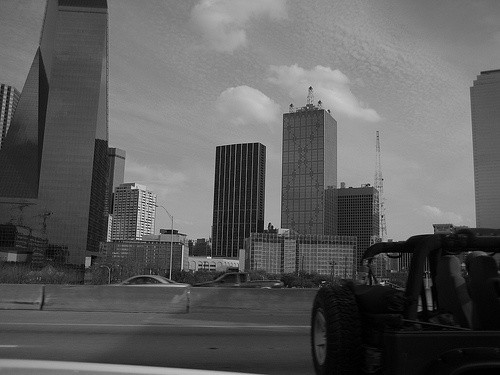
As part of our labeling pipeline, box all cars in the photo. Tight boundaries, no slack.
[118,273,192,287]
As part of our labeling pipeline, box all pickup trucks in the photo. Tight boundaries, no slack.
[195,270,285,288]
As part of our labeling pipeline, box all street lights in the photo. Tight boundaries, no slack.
[100,264,119,284]
[153,204,174,281]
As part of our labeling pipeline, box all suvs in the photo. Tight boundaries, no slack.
[310,223,499,375]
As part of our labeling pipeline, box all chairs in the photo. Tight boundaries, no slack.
[451,255,500,332]
